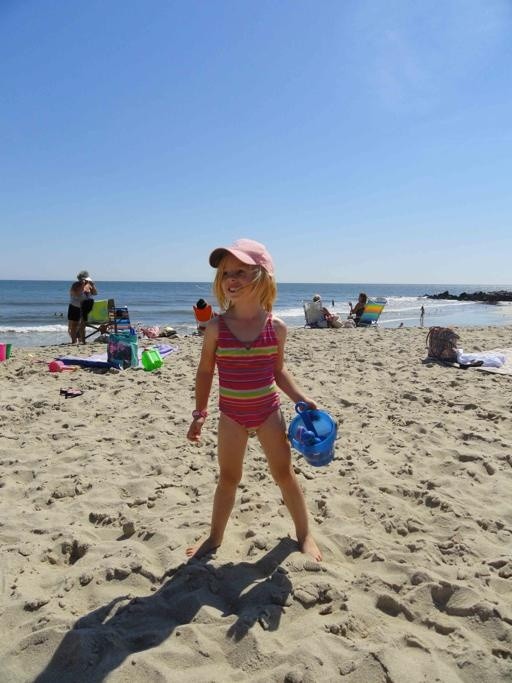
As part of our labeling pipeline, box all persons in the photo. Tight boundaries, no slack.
[186,239,321,563]
[312,293,367,323]
[196,298,208,309]
[420,305,425,320]
[67,270,98,344]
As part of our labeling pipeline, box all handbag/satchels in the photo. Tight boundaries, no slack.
[426,325,459,363]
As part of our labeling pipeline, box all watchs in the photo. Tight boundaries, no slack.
[192,410,208,418]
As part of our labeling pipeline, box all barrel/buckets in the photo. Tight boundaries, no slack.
[286,409,340,468]
[0,342,7,361]
[6,344,13,359]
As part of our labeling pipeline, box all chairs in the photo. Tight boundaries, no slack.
[355,300,385,327]
[193,304,215,331]
[80,296,131,340]
[301,300,330,327]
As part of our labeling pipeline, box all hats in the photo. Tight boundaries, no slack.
[76,270,92,283]
[209,239,274,276]
